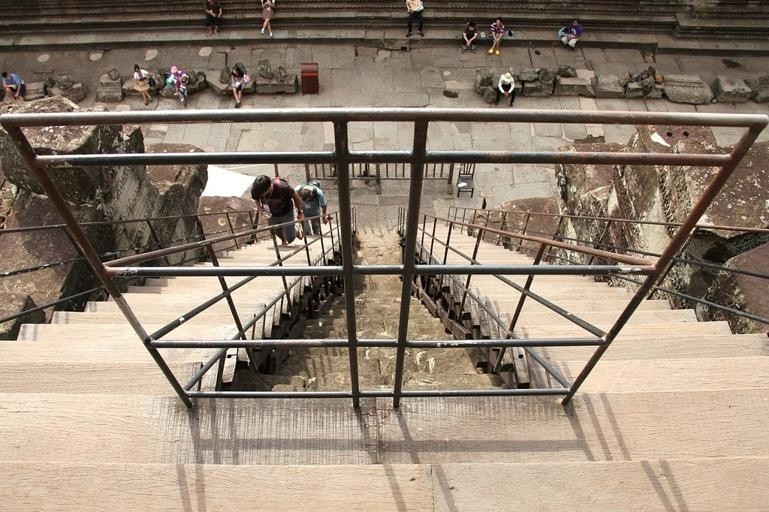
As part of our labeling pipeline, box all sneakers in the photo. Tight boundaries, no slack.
[235,100,241,107]
[145,96,152,104]
[259,30,273,39]
[488,48,501,55]
[295,224,303,240]
[406,31,424,37]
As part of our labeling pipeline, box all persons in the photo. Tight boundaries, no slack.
[1,71,26,101]
[493,71,516,107]
[294,183,328,237]
[175,71,188,97]
[203,0,223,37]
[165,65,189,108]
[560,18,585,53]
[488,16,505,57]
[557,25,578,48]
[231,65,245,109]
[260,0,276,38]
[250,174,305,247]
[133,63,153,106]
[461,18,479,54]
[406,0,425,38]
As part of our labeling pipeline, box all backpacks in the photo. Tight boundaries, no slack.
[272,177,289,195]
[298,181,321,200]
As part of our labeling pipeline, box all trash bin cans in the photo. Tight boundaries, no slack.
[301,63,318,94]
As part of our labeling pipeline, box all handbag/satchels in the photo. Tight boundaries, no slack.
[243,74,252,83]
[149,77,156,87]
[165,76,174,88]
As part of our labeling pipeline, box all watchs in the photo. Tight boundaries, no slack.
[297,208,304,214]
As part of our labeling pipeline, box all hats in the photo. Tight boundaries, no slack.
[171,65,177,72]
